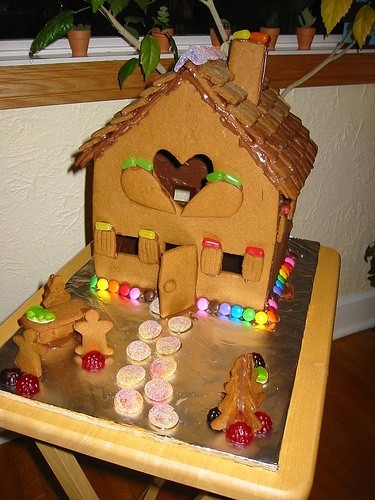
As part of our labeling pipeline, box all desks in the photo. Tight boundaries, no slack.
[18,296,89,345]
[0,238,342,500]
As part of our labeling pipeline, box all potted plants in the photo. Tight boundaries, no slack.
[296,7,317,50]
[260,11,280,51]
[151,6,174,54]
[210,18,231,49]
[67,23,92,57]
